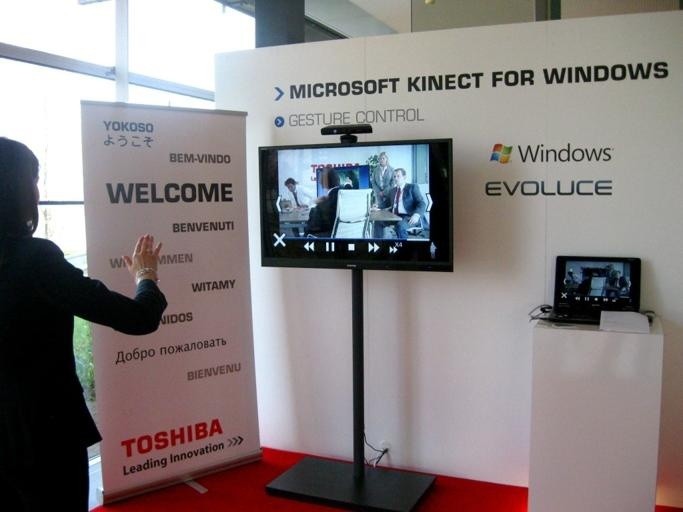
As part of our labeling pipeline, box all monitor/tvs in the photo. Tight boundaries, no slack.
[257,139,455,274]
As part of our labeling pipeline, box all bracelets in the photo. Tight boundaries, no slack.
[134,266,160,287]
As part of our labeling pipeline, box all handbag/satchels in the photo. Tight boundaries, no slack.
[308,208,333,232]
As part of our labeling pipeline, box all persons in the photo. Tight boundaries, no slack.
[563,265,629,298]
[370,151,395,210]
[279,178,317,238]
[0,135,167,512]
[305,167,344,237]
[373,168,426,240]
[342,170,358,188]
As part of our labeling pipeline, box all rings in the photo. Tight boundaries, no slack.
[145,248,152,254]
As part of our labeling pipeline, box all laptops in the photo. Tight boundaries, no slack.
[547,255,642,326]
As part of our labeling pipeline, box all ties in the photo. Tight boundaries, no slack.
[293,191,300,207]
[394,188,400,214]
